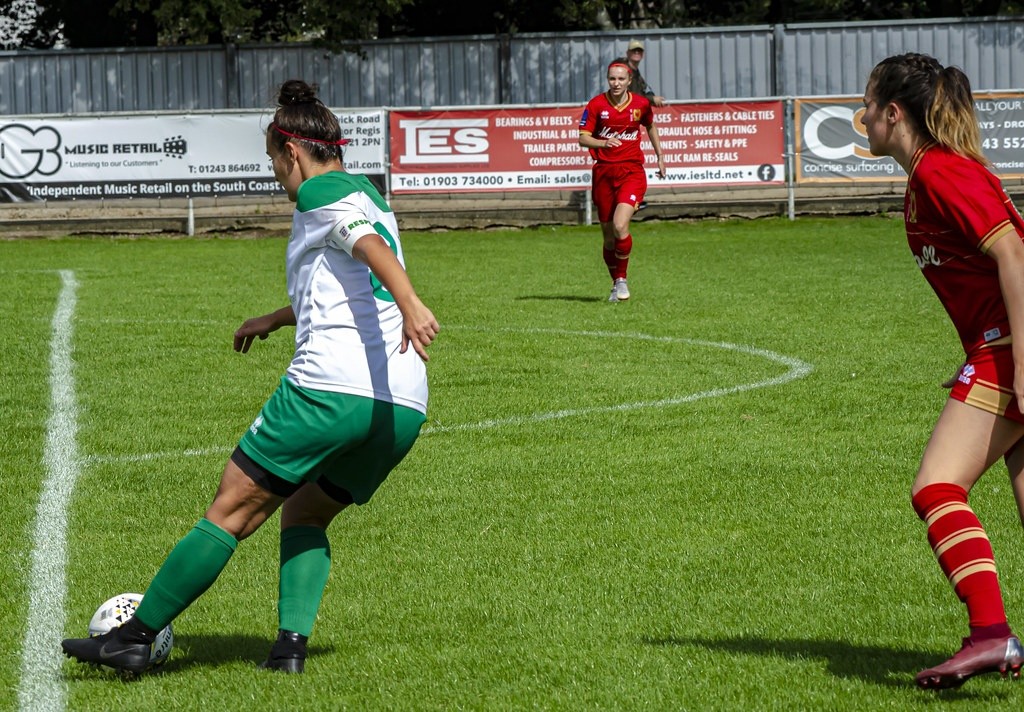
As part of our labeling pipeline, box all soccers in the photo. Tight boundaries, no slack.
[90,593,174,667]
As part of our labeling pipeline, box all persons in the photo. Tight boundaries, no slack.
[59,81,443,684]
[577,58,669,306]
[859,52,1024,692]
[623,42,667,106]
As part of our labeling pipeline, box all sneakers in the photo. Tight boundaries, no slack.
[61,624,151,682]
[258,628,310,674]
[609,286,620,302]
[615,277,630,299]
[913,635,1024,691]
